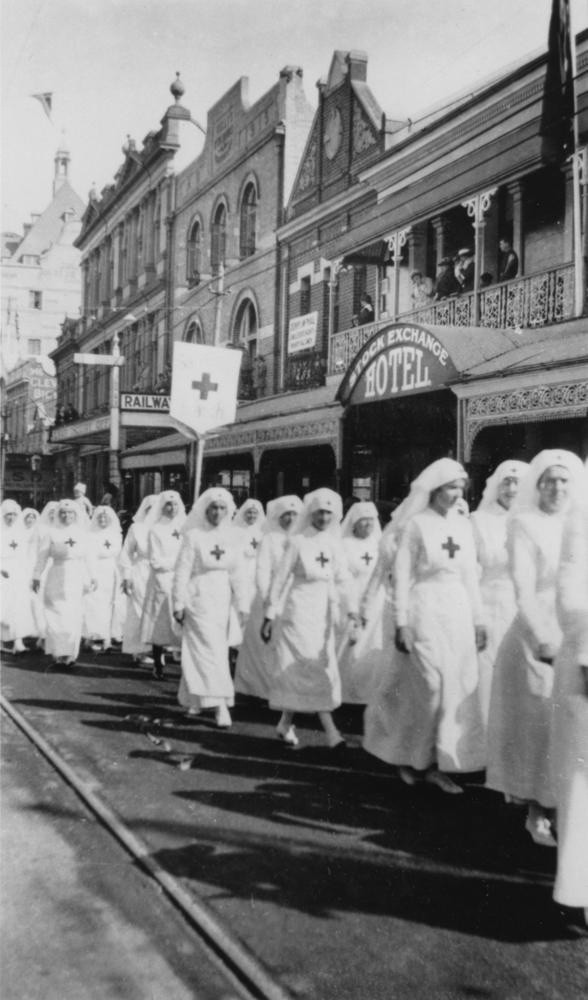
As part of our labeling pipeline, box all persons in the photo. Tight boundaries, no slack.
[51,235,518,427]
[0,447,588,915]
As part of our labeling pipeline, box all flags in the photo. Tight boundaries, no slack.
[539,1,572,176]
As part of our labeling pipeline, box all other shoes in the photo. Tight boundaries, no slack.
[400,770,415,785]
[132,654,154,664]
[275,723,298,744]
[328,732,347,751]
[13,647,29,655]
[524,812,557,848]
[171,654,181,662]
[103,642,112,651]
[85,641,101,651]
[423,773,465,795]
[160,653,166,666]
[188,708,201,714]
[152,667,164,681]
[215,707,232,727]
